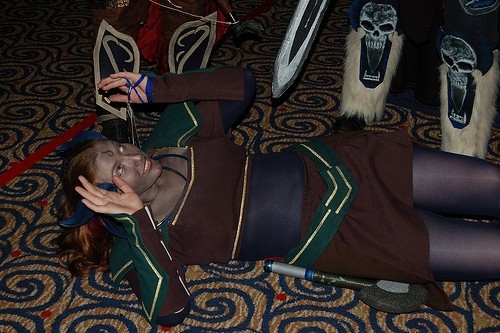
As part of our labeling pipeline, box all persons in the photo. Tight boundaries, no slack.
[55,69,500,323]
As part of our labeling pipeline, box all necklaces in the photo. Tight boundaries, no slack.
[152,152,190,182]
